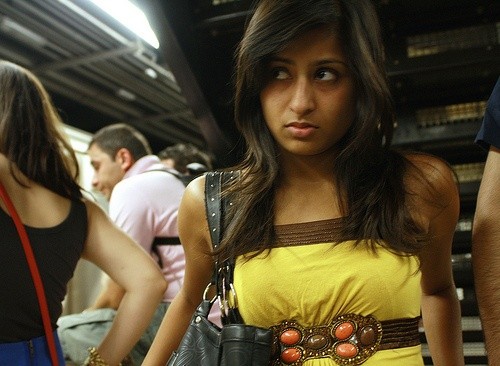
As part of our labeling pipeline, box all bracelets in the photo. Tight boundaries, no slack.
[87,346,123,366]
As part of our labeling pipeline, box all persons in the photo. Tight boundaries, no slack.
[81,124,224,366]
[140,0,465,366]
[0,58,167,366]
[472,78,500,366]
[158,143,212,177]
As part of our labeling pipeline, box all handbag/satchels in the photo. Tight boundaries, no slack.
[168,168,276,363]
